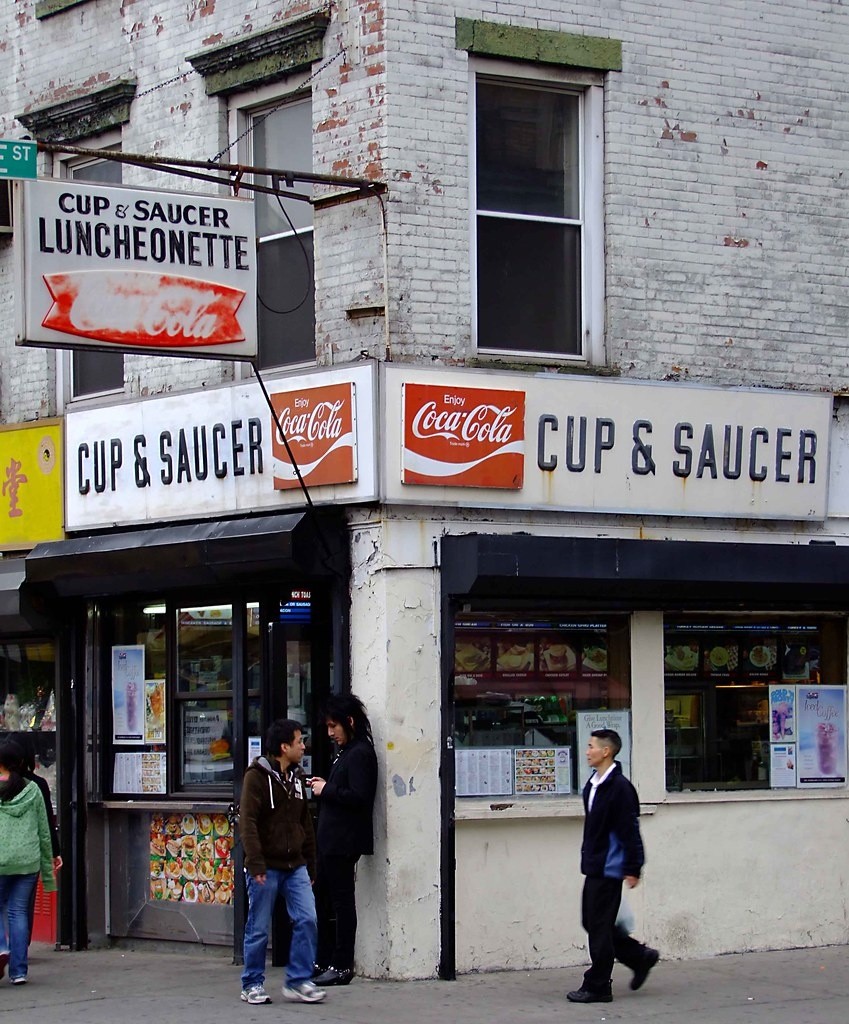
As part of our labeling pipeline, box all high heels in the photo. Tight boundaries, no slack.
[311,965,354,984]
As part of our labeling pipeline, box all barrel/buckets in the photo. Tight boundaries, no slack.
[454,684,477,699]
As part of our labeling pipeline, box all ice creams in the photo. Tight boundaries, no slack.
[776,702,788,739]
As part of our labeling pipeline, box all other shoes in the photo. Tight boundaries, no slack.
[11,977,28,983]
[0,952,10,980]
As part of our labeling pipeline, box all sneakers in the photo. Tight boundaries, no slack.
[241,985,272,1004]
[282,980,326,1001]
[567,986,613,1003]
[630,949,659,990]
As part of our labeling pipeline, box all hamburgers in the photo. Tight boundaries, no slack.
[549,644,567,668]
[462,647,481,664]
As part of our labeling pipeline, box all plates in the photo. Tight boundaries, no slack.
[182,813,229,836]
[709,647,729,667]
[583,647,607,672]
[749,645,771,667]
[166,841,214,902]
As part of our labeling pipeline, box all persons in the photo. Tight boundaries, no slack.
[306,693,378,984]
[0,731,63,983]
[241,720,327,1003]
[568,730,659,1003]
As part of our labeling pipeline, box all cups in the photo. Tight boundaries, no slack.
[817,722,839,777]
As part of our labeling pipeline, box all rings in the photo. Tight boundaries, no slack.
[312,789,315,791]
